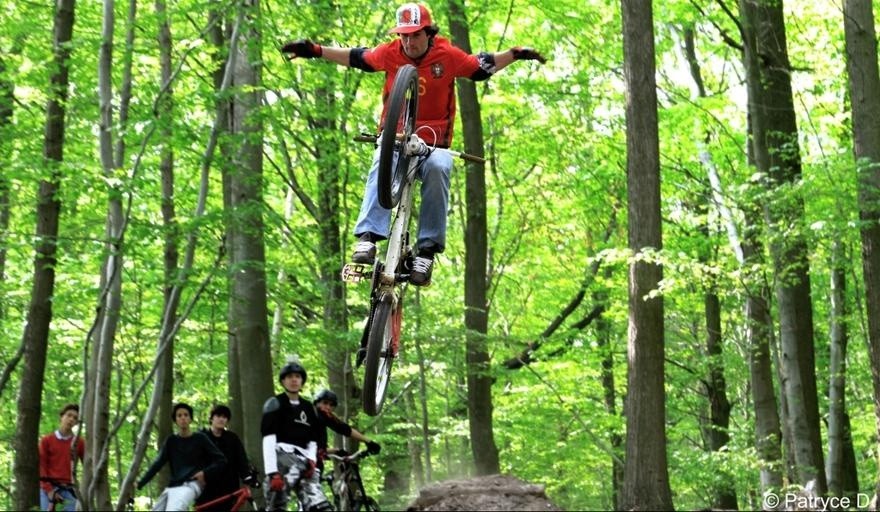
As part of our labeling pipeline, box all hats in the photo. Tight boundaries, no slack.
[388,3,432,34]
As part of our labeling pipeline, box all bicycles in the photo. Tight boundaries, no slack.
[39,472,82,511]
[193,466,265,510]
[341,67,488,416]
[262,444,382,512]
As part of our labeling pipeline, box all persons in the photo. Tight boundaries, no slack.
[197,403,251,505]
[281,2,548,285]
[257,361,335,512]
[311,388,379,484]
[40,403,85,512]
[130,402,227,511]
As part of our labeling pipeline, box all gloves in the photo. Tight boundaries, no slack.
[270,473,288,490]
[334,448,352,459]
[316,452,325,472]
[512,46,546,65]
[364,440,380,455]
[282,39,322,60]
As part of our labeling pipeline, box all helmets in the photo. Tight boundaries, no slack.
[313,389,337,408]
[281,362,306,386]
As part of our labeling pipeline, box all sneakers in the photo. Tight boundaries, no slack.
[352,232,377,264]
[408,249,434,286]
